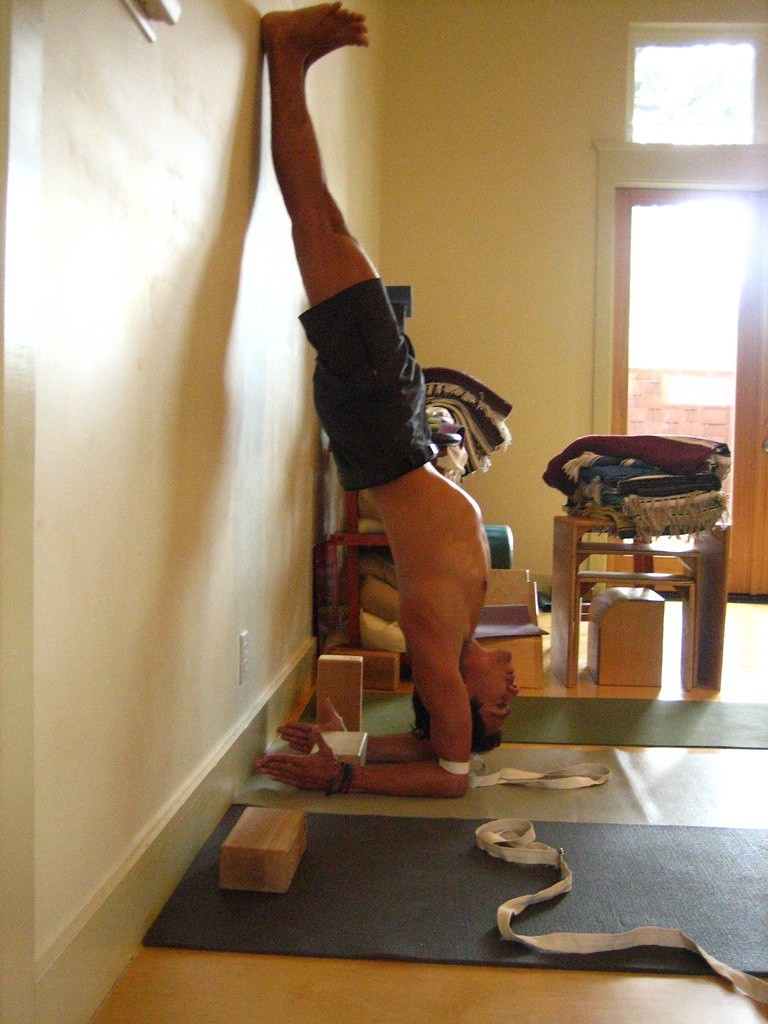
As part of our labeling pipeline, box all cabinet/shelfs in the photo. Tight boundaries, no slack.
[328,441,446,665]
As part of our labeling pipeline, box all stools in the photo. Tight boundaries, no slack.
[575,572,696,691]
[550,515,731,692]
[571,541,704,689]
[588,585,665,688]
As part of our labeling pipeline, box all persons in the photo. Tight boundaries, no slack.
[257,2,517,798]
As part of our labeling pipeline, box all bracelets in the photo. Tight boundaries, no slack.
[318,761,353,797]
